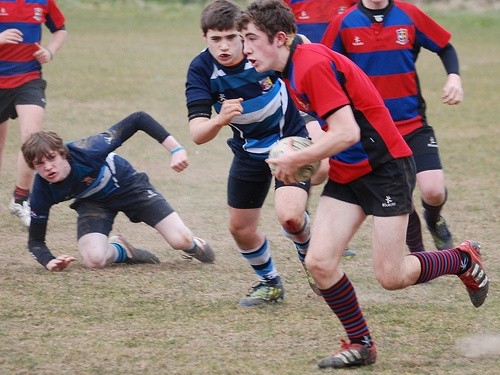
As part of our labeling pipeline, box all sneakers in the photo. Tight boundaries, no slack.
[185,239,215,263]
[8,197,31,227]
[240,276,284,306]
[423,211,453,252]
[299,258,323,297]
[342,249,355,259]
[454,240,489,308]
[108,235,160,265]
[317,339,376,369]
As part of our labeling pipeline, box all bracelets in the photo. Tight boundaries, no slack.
[47,48,53,60]
[169,146,185,154]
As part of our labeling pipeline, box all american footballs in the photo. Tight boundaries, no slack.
[268,136,320,182]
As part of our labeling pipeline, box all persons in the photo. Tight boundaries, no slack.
[235,0,489,369]
[282,0,357,186]
[185,0,322,307]
[21,111,215,271]
[321,0,463,253]
[0,0,67,229]
